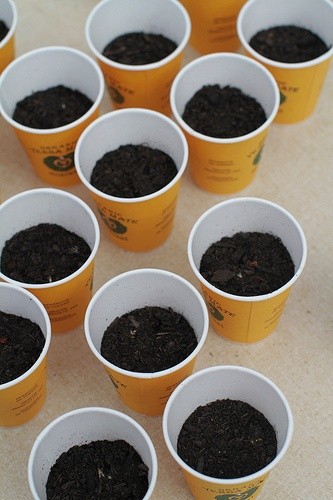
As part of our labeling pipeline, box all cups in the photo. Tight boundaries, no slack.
[236,0,333,125]
[161,366,293,500]
[84,269,209,416]
[178,0,252,55]
[0,283,51,426]
[0,46,103,188]
[170,52,280,194]
[0,189,100,334]
[0,0,18,75]
[74,109,189,253]
[28,407,158,500]
[187,197,307,344]
[86,0,191,118]
